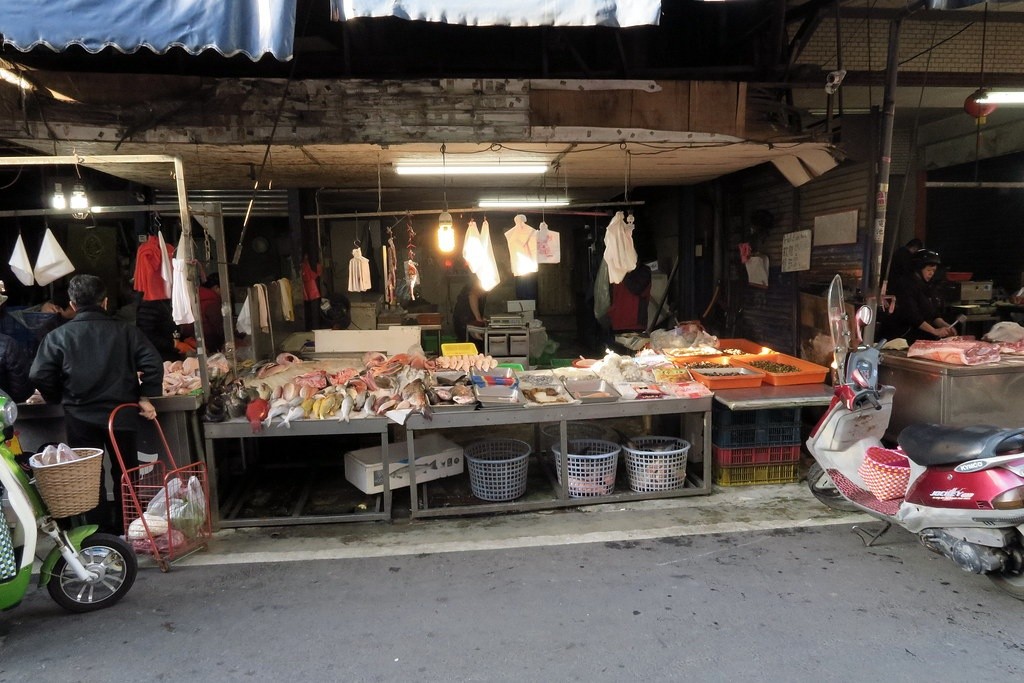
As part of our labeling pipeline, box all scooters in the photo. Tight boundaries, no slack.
[804,274,1023,604]
[0,386,139,615]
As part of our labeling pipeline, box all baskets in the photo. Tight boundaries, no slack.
[29,447,104,518]
[462,438,531,501]
[542,423,690,500]
[857,445,912,501]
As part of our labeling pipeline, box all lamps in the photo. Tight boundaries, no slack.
[392,162,551,176]
[975,0,1024,105]
[476,197,571,208]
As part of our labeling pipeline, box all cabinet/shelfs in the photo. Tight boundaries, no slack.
[466,320,529,370]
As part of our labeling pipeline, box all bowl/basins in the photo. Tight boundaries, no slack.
[572,358,596,367]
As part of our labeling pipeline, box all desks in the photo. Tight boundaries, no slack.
[405,347,714,519]
[202,355,391,532]
[416,325,442,358]
[614,332,835,467]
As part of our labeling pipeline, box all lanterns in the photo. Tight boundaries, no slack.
[963,89,996,125]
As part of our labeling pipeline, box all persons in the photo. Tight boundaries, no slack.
[452,282,488,350]
[885,239,956,346]
[112,254,247,360]
[26,275,164,534]
[0,276,78,405]
[608,264,652,339]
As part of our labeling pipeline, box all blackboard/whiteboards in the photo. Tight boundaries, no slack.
[781,229,811,272]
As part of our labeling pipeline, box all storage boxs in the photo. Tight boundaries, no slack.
[943,281,994,301]
[712,406,803,427]
[711,442,800,467]
[345,432,464,494]
[711,460,800,486]
[518,311,534,321]
[346,294,384,330]
[507,300,535,313]
[712,423,801,448]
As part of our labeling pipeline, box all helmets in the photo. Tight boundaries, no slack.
[914,249,941,271]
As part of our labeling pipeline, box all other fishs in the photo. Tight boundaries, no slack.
[226,375,476,434]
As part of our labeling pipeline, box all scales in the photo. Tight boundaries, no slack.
[491,313,523,329]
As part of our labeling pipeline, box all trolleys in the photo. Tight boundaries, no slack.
[109,403,212,574]
